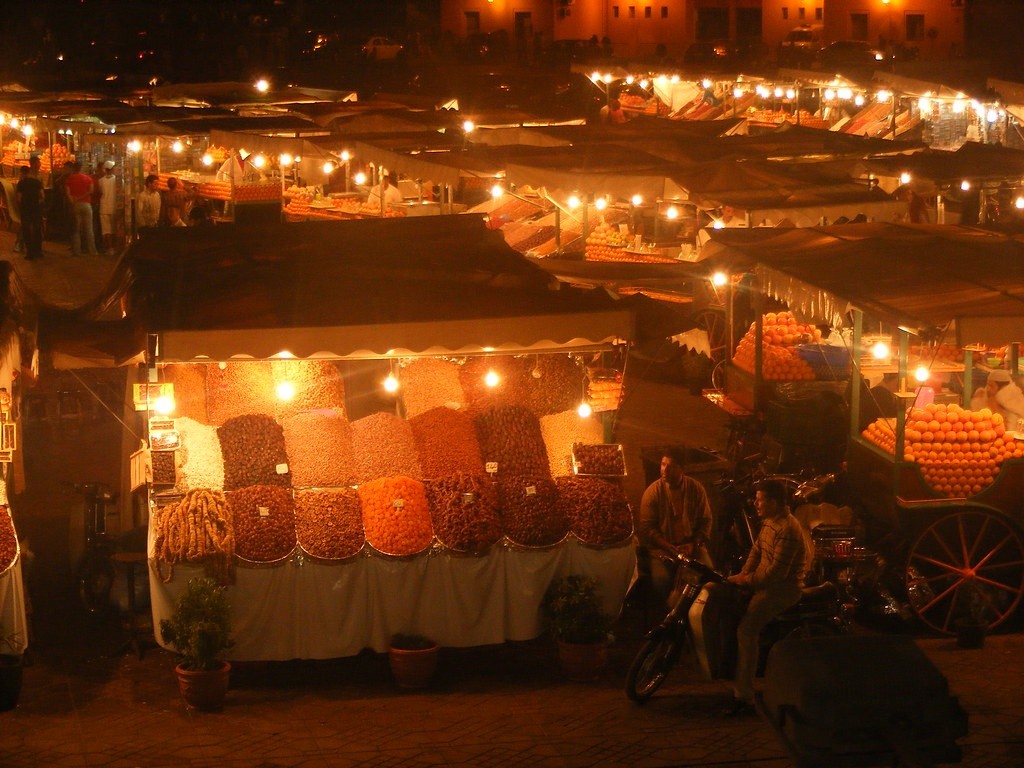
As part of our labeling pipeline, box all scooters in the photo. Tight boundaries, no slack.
[624,552,845,704]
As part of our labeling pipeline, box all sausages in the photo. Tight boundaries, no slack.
[151,487,236,589]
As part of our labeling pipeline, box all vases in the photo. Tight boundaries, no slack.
[0,655,25,710]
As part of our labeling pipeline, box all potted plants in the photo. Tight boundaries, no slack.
[160,575,231,711]
[954,577,988,647]
[552,573,613,679]
[386,633,439,688]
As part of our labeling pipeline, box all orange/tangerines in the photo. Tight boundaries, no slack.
[862,403,1024,498]
[584,245,681,263]
[733,311,825,381]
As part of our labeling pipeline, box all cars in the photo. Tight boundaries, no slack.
[816,40,896,66]
[683,41,736,67]
[351,32,405,61]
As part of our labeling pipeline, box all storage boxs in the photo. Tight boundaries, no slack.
[797,344,850,367]
[950,369,989,393]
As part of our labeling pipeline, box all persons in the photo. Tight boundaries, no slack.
[599,99,626,123]
[640,448,815,716]
[0,135,258,259]
[972,369,1018,433]
[708,203,747,228]
[695,84,720,107]
[861,372,904,428]
[369,164,403,205]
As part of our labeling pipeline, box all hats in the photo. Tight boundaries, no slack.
[167,177,177,190]
[104,159,116,170]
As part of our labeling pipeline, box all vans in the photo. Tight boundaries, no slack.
[779,24,823,57]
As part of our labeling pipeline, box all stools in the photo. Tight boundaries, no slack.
[111,552,158,661]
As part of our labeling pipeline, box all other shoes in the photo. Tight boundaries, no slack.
[721,698,744,716]
